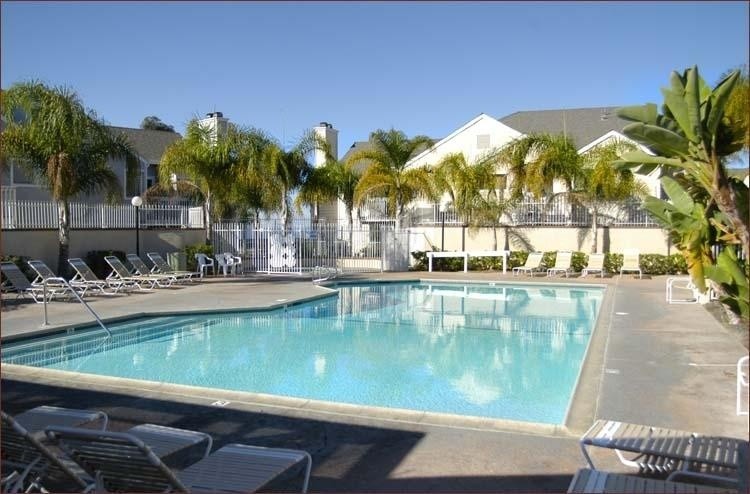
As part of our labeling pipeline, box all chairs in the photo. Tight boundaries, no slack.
[3,406,110,489]
[0,411,214,491]
[43,418,312,493]
[513,249,643,279]
[0,252,242,303]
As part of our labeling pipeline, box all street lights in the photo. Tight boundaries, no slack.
[131,196,142,256]
[439,205,448,251]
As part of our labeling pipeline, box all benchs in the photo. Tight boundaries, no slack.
[559,419,749,494]
